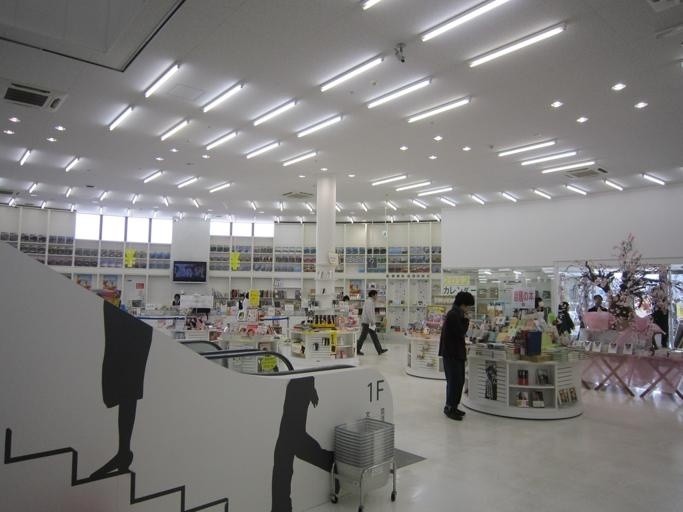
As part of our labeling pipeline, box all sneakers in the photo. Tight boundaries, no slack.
[356,350,364,355]
[454,408,465,416]
[442,406,463,421]
[377,348,389,355]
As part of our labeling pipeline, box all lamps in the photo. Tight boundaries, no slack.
[7,0,667,223]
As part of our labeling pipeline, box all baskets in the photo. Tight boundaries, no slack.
[334,416,395,495]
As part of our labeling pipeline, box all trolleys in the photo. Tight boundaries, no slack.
[328,458,399,512]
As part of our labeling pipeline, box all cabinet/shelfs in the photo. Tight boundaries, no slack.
[1,230,682,421]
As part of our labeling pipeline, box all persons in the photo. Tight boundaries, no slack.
[588,295,607,312]
[355,290,388,355]
[532,297,548,325]
[556,302,575,335]
[439,291,475,420]
[173,294,180,306]
[242,292,249,319]
[647,307,678,348]
[238,294,245,309]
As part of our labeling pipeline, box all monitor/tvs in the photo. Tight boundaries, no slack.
[173,261,207,283]
